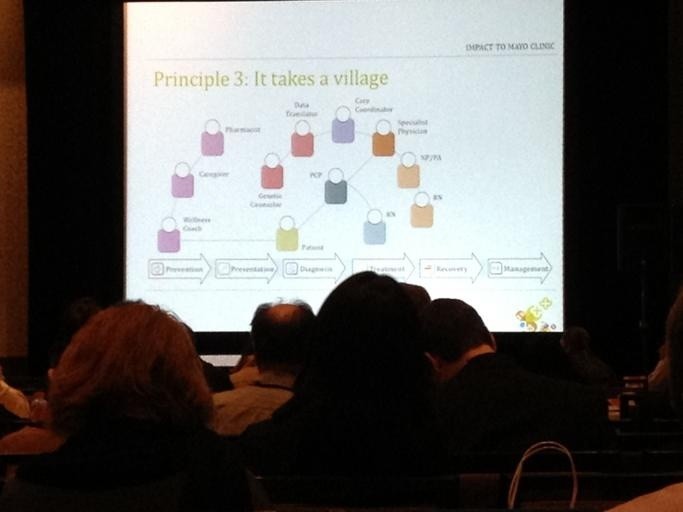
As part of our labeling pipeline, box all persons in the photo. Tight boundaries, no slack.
[1,268,681,511]
